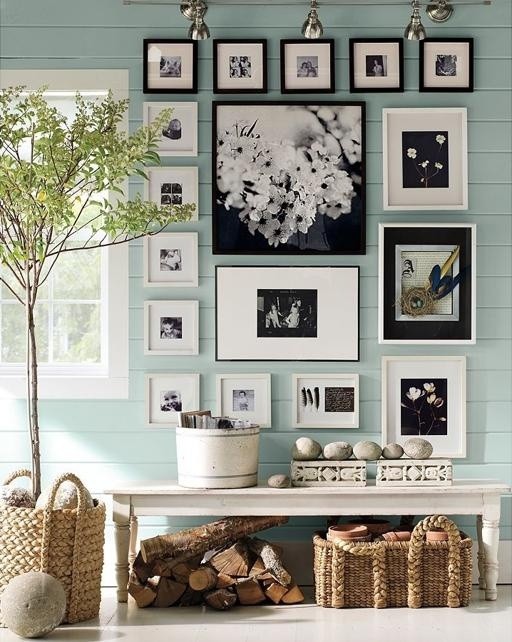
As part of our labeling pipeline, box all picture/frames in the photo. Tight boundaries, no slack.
[280,38,334,94]
[213,39,267,93]
[349,37,404,93]
[143,101,198,157]
[378,222,476,344]
[215,265,360,362]
[382,108,468,210]
[143,38,197,94]
[217,374,271,429]
[381,355,466,458]
[292,374,359,428]
[143,231,198,288]
[212,101,366,254]
[418,37,473,92]
[144,300,200,355]
[143,373,200,429]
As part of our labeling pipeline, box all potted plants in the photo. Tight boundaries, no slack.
[0,84,198,624]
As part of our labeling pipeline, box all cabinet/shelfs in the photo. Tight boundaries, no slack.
[106,479,512,602]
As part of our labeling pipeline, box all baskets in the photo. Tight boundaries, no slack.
[0,472,107,629]
[312,515,474,609]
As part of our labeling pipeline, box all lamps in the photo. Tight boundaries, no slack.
[403,15,426,40]
[189,14,210,40]
[301,12,325,39]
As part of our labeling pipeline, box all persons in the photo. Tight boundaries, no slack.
[371,59,383,77]
[238,390,250,412]
[286,305,301,328]
[161,320,179,338]
[159,390,182,413]
[161,119,182,140]
[297,61,315,77]
[230,56,252,78]
[270,304,283,328]
